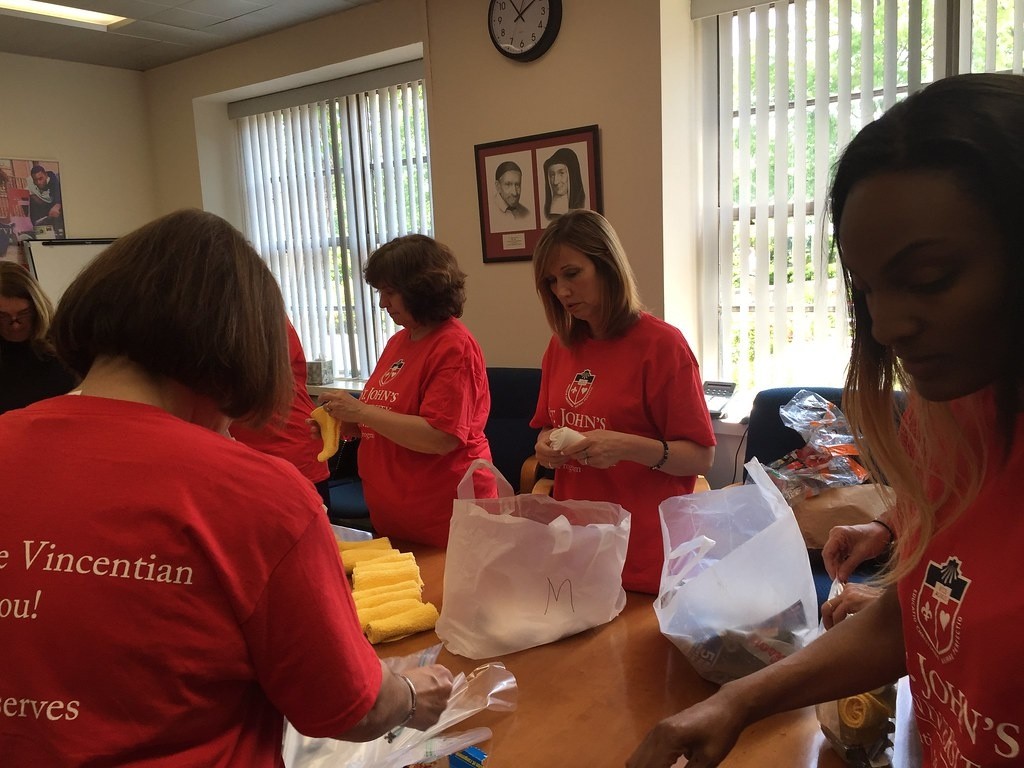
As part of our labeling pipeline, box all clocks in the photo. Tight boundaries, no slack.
[488,0,563,62]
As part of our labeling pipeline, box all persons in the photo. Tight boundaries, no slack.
[225,314,332,526]
[625,74,1024,768]
[531,208,718,601]
[306,234,500,552]
[821,507,899,632]
[0,261,82,416]
[0,207,458,767]
[21,164,64,238]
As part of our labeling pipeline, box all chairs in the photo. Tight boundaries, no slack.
[723,387,904,624]
[484,366,554,496]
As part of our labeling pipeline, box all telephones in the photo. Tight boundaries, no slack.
[701,380,736,419]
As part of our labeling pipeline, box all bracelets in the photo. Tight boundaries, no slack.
[871,520,896,548]
[384,672,416,744]
[650,440,669,470]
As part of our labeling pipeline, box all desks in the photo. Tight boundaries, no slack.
[332,522,923,768]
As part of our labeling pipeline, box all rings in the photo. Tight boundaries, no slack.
[826,601,836,611]
[327,402,332,411]
[585,457,588,465]
[548,461,552,469]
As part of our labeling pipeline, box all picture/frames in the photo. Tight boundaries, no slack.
[473,123,602,264]
